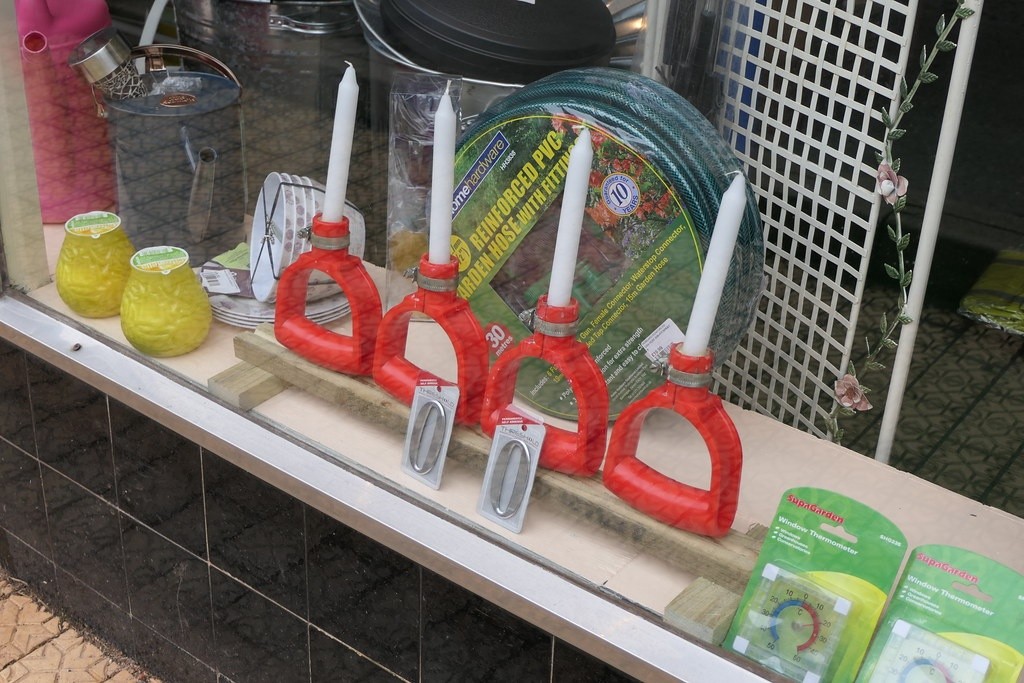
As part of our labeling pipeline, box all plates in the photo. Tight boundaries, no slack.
[204,289,355,332]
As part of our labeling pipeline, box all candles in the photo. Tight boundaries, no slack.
[428,81,456,267]
[321,60,360,221]
[546,119,593,308]
[681,170,747,356]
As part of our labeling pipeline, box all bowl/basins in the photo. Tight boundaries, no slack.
[248,170,366,306]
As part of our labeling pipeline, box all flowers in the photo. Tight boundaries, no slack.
[824,0,975,446]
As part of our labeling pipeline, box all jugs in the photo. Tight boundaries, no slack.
[69,26,247,266]
